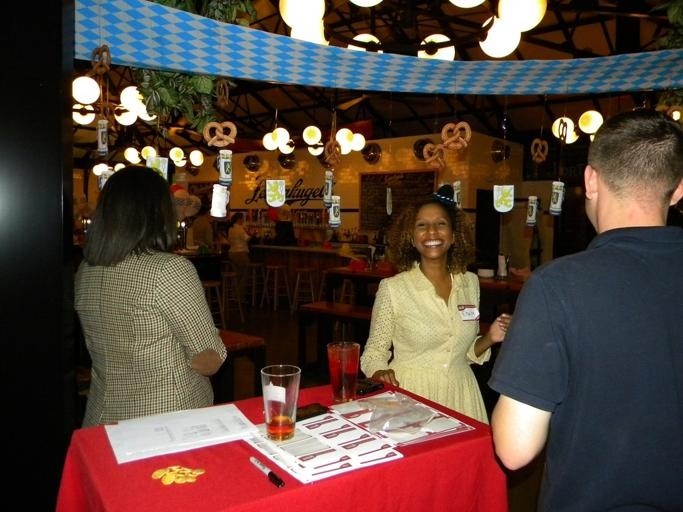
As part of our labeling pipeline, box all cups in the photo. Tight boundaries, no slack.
[326,341,361,403]
[260,365,302,441]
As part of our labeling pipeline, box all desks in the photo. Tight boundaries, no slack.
[321,260,527,315]
[51,376,509,511]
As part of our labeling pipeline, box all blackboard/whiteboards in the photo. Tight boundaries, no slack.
[358,169,438,230]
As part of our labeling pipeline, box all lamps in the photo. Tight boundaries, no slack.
[75,0,602,180]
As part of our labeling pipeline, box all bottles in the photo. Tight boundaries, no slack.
[340,227,359,242]
[296,211,320,224]
[496,255,507,280]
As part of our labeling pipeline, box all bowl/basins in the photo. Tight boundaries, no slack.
[477,269,494,278]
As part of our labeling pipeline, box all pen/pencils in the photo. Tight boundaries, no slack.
[250,457,285,488]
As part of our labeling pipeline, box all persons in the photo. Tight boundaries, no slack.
[226,212,252,304]
[489,106,683,512]
[361,186,512,426]
[73,164,228,428]
[270,204,298,246]
[192,206,213,245]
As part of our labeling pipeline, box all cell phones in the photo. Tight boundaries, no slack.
[358,382,386,395]
[297,403,325,421]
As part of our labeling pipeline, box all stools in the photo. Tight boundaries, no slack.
[294,298,490,366]
[201,257,354,332]
[208,329,267,404]
